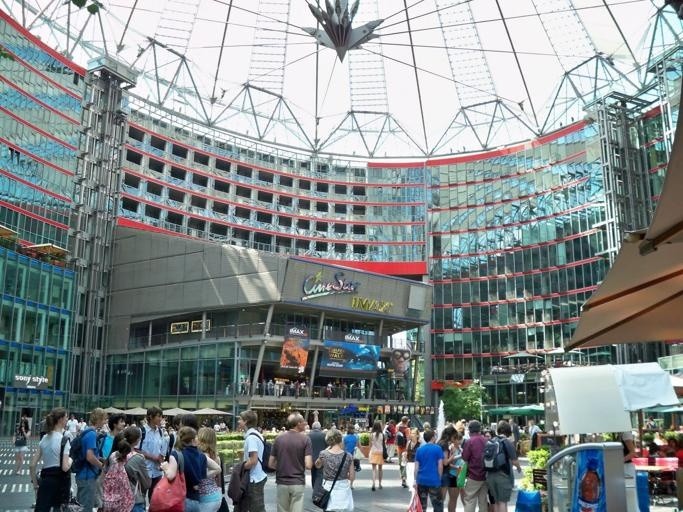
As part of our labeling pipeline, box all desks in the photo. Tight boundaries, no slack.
[634,465,671,505]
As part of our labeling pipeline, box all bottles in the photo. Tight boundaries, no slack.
[578,459,602,512]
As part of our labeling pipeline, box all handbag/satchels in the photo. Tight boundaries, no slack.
[312,480,330,510]
[383,445,388,459]
[217,497,230,512]
[150,471,187,512]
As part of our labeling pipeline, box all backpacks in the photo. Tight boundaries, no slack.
[69,436,83,473]
[481,438,502,472]
[101,460,136,512]
[262,439,276,474]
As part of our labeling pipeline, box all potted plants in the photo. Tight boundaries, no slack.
[358,432,371,459]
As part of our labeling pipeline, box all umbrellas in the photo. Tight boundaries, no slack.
[486,404,545,426]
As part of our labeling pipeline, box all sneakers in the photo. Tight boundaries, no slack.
[16,472,22,475]
[402,482,408,488]
[378,486,383,489]
[372,487,376,491]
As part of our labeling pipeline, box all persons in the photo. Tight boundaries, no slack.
[313,427,355,511]
[332,410,545,463]
[307,420,326,487]
[11,418,30,476]
[619,409,683,491]
[228,408,267,511]
[390,340,411,373]
[267,412,313,511]
[280,327,308,377]
[30,405,234,511]
[369,424,385,492]
[224,374,407,401]
[395,416,521,511]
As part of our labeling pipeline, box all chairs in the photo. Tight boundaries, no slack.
[655,456,679,497]
[631,458,649,466]
